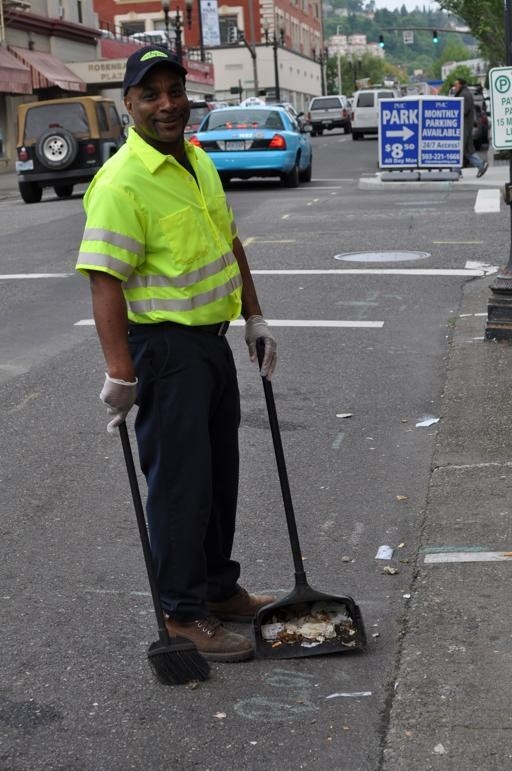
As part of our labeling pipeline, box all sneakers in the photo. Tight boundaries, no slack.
[476,162,489,178]
[459,172,463,179]
[202,585,276,626]
[162,610,255,665]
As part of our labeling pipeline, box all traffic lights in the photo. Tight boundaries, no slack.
[433,31,438,44]
[379,35,384,48]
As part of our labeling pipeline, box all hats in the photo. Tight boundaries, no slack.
[121,43,190,97]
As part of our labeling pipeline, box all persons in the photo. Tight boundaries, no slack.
[452,78,489,178]
[76,42,280,665]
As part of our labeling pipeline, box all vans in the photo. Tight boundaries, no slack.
[448,86,486,107]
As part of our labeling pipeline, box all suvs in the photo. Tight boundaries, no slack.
[15,95,130,204]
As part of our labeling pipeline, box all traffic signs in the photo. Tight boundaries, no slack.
[379,97,465,169]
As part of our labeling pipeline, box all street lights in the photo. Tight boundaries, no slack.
[336,25,344,95]
[311,40,329,96]
[262,16,286,101]
[347,55,363,90]
[162,0,193,66]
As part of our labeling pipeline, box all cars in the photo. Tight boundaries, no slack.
[185,89,399,187]
[472,106,487,151]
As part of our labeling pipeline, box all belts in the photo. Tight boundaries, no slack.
[128,320,231,339]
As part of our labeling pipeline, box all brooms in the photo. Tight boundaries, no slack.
[117,420,210,687]
